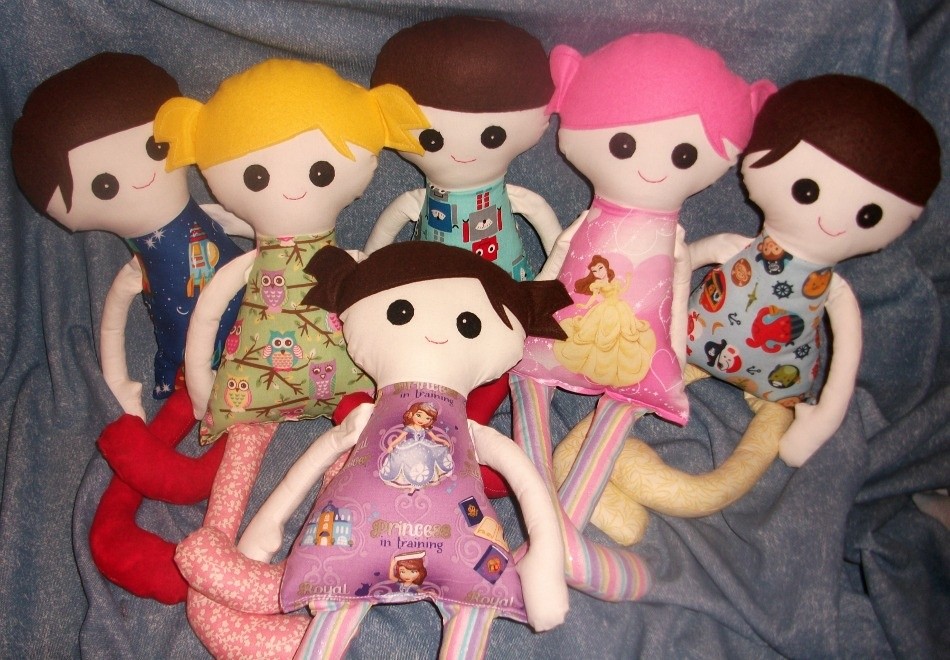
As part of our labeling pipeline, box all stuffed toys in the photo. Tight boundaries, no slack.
[365,10,566,287]
[9,49,255,607]
[505,29,782,607]
[234,238,573,660]
[149,55,433,660]
[548,70,945,547]
